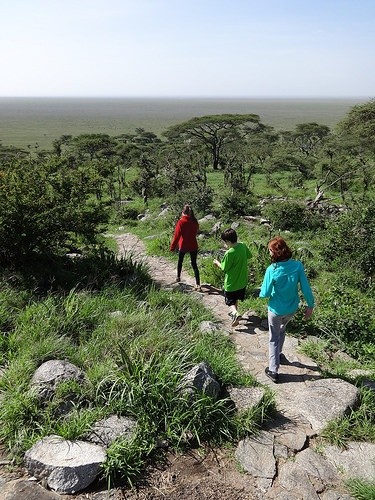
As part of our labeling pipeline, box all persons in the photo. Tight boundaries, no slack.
[169,205,202,292]
[213,228,252,327]
[259,236,314,381]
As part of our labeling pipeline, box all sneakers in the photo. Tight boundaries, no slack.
[192,286,202,291]
[174,278,180,282]
[230,311,242,327]
[228,312,234,317]
[264,367,279,383]
